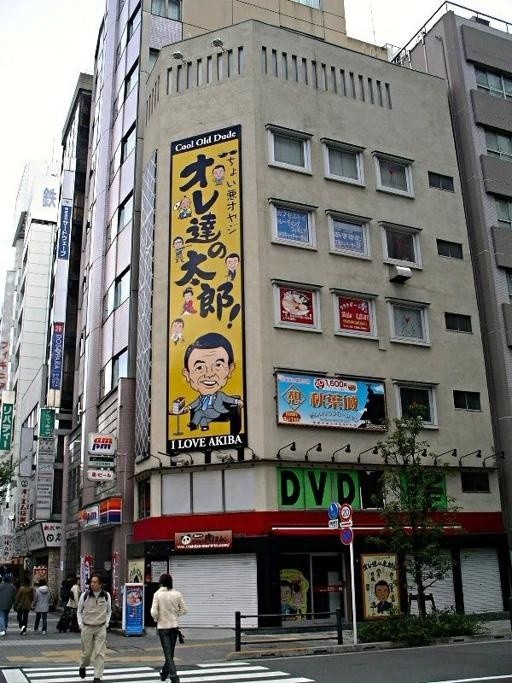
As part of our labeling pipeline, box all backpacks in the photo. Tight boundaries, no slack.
[62,584,74,602]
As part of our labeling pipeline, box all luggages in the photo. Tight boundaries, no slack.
[57,614,71,633]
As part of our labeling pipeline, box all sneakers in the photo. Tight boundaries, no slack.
[21,626,26,635]
[0,631,6,636]
[34,630,46,635]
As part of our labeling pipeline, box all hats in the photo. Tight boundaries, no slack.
[4,576,13,583]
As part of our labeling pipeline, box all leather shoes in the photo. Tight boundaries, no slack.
[79,668,85,678]
[160,669,166,681]
[93,680,102,683]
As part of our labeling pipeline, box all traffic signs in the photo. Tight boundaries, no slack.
[339,504,352,521]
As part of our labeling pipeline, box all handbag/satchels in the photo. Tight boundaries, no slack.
[178,630,184,644]
[14,602,23,612]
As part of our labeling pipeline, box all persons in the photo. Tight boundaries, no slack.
[0,566,21,590]
[149,573,188,683]
[13,577,33,635]
[58,575,82,633]
[32,579,51,636]
[0,577,18,637]
[77,573,112,683]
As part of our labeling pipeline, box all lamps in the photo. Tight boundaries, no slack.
[210,38,226,52]
[172,52,186,65]
[277,442,505,468]
[142,441,256,468]
